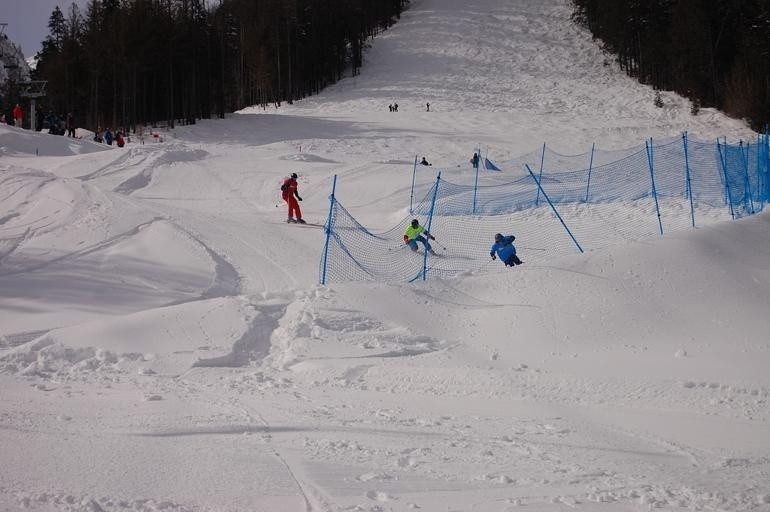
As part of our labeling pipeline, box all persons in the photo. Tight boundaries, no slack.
[473,153,478,168]
[67,111,78,139]
[404,218,438,256]
[13,103,23,128]
[280,172,307,224]
[420,156,428,165]
[389,104,392,111]
[58,114,66,135]
[427,103,430,111]
[489,232,524,267]
[103,127,113,145]
[35,103,45,131]
[0,113,8,125]
[394,103,398,112]
[392,106,394,112]
[113,131,125,148]
[95,126,103,143]
[45,107,58,134]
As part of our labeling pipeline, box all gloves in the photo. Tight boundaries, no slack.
[490,250,496,260]
[428,234,436,242]
[285,184,291,189]
[296,193,302,202]
[405,237,408,245]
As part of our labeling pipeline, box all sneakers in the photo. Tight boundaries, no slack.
[286,217,306,224]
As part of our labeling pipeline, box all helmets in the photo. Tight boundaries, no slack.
[494,233,504,240]
[290,173,298,178]
[412,219,419,223]
